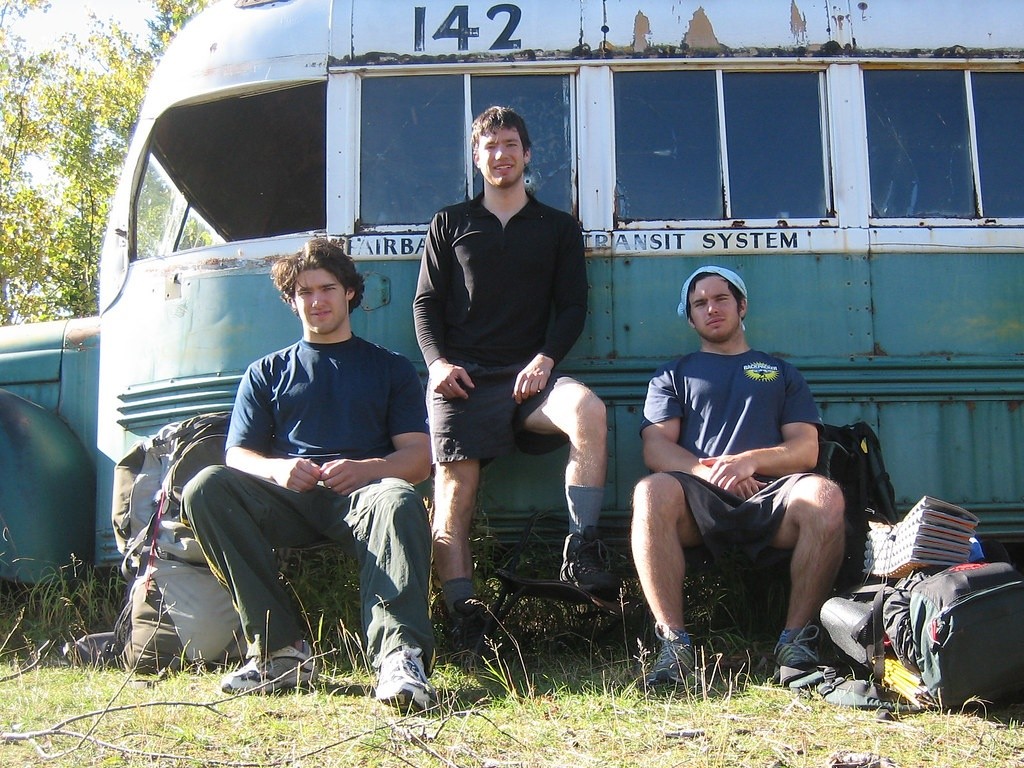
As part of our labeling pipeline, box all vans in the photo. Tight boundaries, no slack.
[0,0,1024,645]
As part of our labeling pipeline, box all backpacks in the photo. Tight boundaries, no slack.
[158,418,232,564]
[874,563,1024,708]
[112,410,263,669]
[817,422,900,566]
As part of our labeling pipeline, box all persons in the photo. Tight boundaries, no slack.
[412,106,621,667]
[180,237,433,713]
[631,265,846,692]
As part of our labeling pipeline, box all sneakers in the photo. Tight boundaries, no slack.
[445,597,503,666]
[221,640,317,694]
[376,645,435,712]
[649,622,693,682]
[559,525,623,592]
[771,620,821,685]
